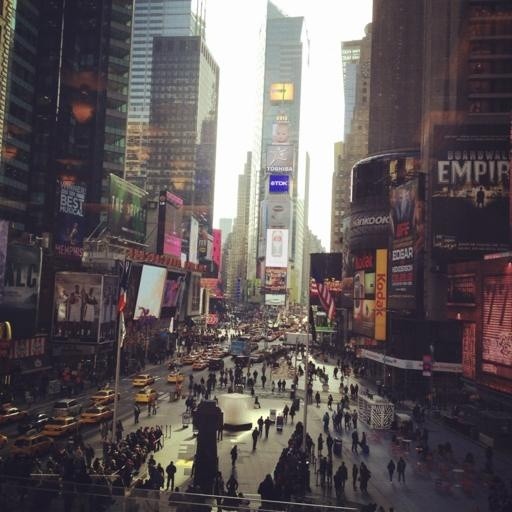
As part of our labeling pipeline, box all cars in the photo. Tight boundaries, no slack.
[42,416,80,439]
[167,371,185,383]
[90,385,121,406]
[17,412,51,435]
[10,428,55,462]
[79,403,113,425]
[0,431,9,447]
[0,402,29,426]
[132,373,154,387]
[135,385,159,403]
[180,315,305,372]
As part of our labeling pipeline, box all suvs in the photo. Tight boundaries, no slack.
[52,398,82,418]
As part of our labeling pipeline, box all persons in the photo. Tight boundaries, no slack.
[2,300,511,510]
[475,186,484,207]
[55,284,117,337]
[272,123,291,145]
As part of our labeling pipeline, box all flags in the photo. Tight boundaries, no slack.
[119,289,126,311]
[308,275,336,321]
